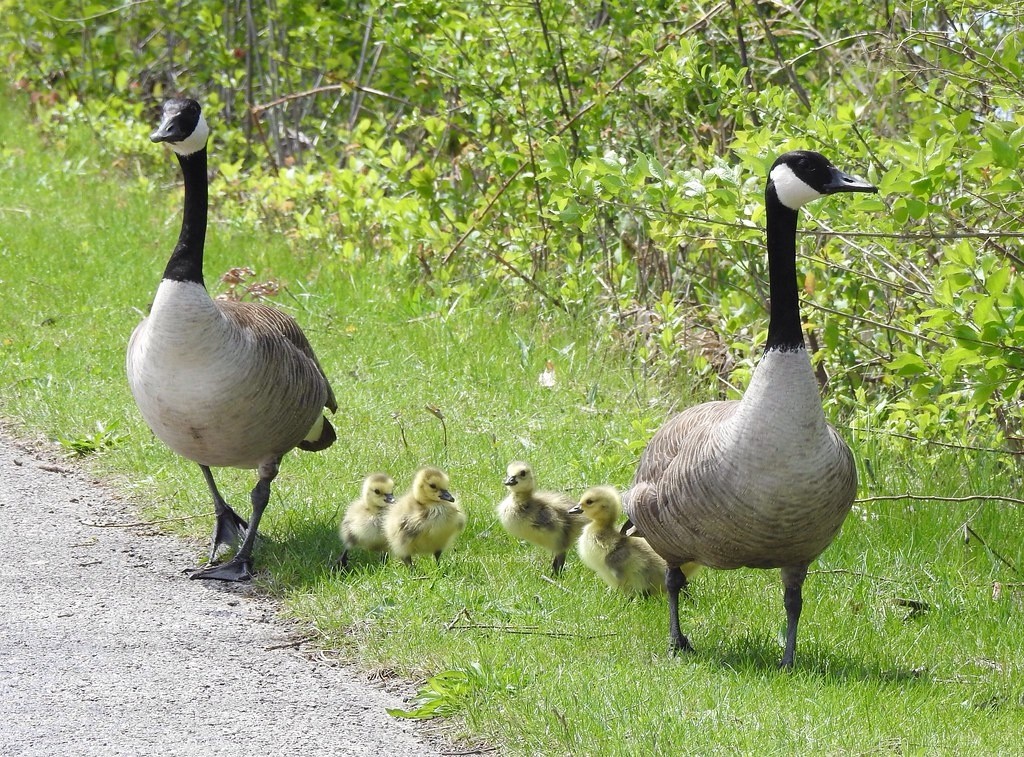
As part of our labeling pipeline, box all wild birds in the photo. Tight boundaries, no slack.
[625,148,879,670]
[339,459,699,602]
[123,97,338,586]
